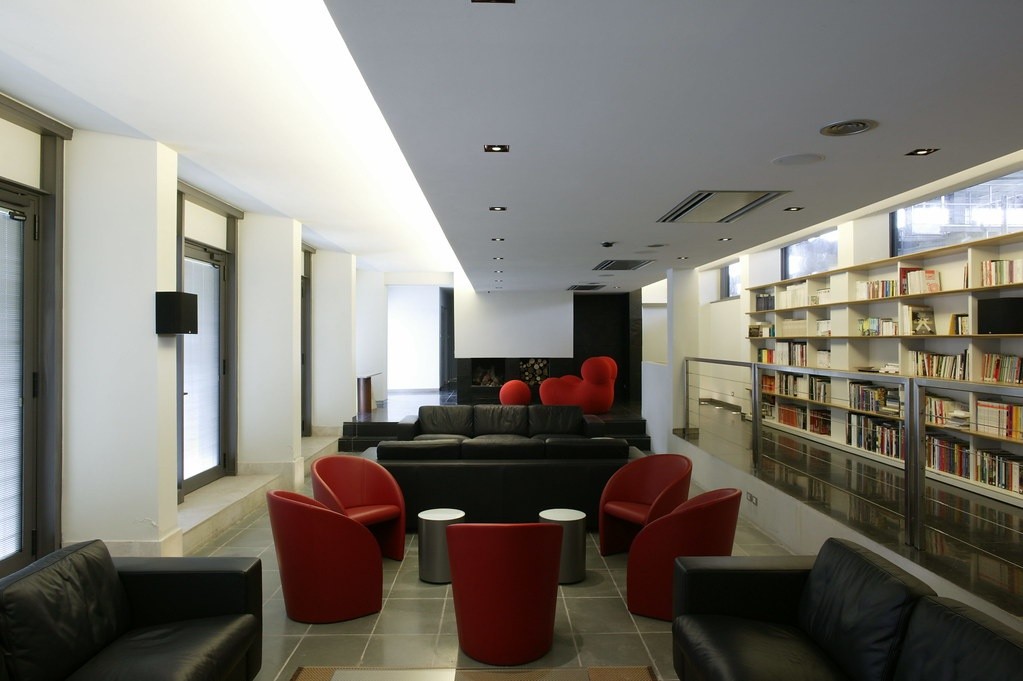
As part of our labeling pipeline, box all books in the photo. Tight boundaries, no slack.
[760,431,1023,618]
[747,257,1023,498]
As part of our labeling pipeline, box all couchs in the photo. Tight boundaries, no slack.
[359,438,648,533]
[394,404,605,440]
[1,538,264,681]
[671,537,1023,681]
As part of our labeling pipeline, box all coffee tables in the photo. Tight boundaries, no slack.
[539,508,586,585]
[291,665,664,681]
[418,508,465,584]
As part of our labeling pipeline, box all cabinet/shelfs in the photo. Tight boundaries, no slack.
[744,228,1023,510]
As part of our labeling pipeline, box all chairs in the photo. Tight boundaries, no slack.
[446,524,563,665]
[627,487,742,622]
[267,489,383,623]
[599,454,692,556]
[311,455,405,561]
